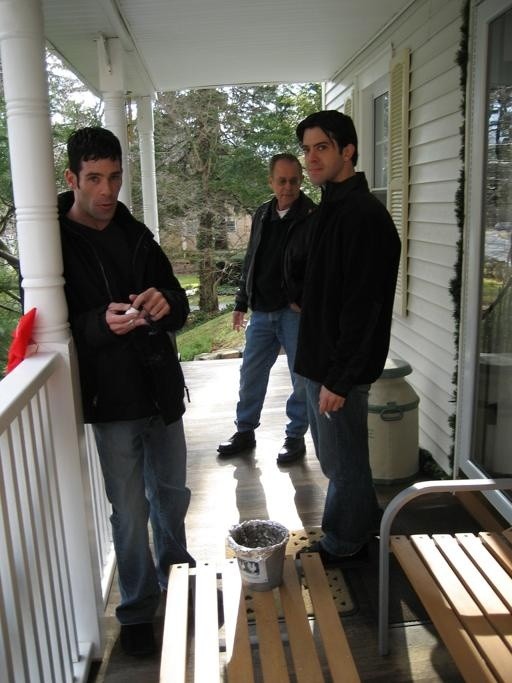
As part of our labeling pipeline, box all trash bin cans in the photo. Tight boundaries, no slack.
[230,519,289,593]
[475,352,512,475]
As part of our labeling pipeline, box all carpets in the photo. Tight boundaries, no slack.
[225,528,357,621]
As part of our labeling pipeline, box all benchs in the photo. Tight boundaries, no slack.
[158,552,359,682]
[379,477,511,683]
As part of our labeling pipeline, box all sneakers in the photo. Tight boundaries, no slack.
[354,507,383,541]
[120,622,155,654]
[297,543,369,566]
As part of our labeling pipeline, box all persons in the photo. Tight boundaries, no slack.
[217,152,317,464]
[289,111,403,571]
[56,125,197,658]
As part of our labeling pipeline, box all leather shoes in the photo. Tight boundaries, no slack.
[278,437,306,464]
[217,430,256,455]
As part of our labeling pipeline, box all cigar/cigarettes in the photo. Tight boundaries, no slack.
[234,324,241,326]
[318,402,331,420]
[127,318,135,326]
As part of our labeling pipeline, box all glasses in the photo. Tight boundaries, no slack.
[274,177,301,185]
